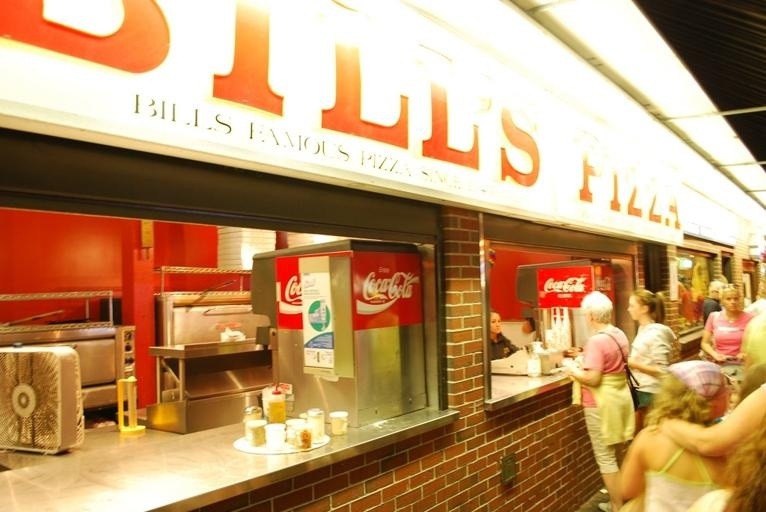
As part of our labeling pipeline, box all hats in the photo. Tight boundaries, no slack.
[671,355,727,396]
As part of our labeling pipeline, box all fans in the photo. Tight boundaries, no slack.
[0,346,86,457]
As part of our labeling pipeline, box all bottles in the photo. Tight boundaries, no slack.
[239,387,325,448]
[529,351,542,377]
[552,306,573,348]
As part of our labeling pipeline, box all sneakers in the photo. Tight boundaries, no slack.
[597,500,623,512]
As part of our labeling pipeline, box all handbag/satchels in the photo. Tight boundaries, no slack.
[628,385,640,412]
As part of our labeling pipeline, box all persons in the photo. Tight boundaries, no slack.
[660,376,766,511]
[517,317,541,349]
[702,278,723,326]
[559,288,639,512]
[698,285,754,363]
[737,308,766,372]
[488,310,523,365]
[625,287,677,434]
[607,358,733,512]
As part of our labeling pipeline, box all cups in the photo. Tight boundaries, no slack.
[532,341,543,353]
[330,411,348,435]
